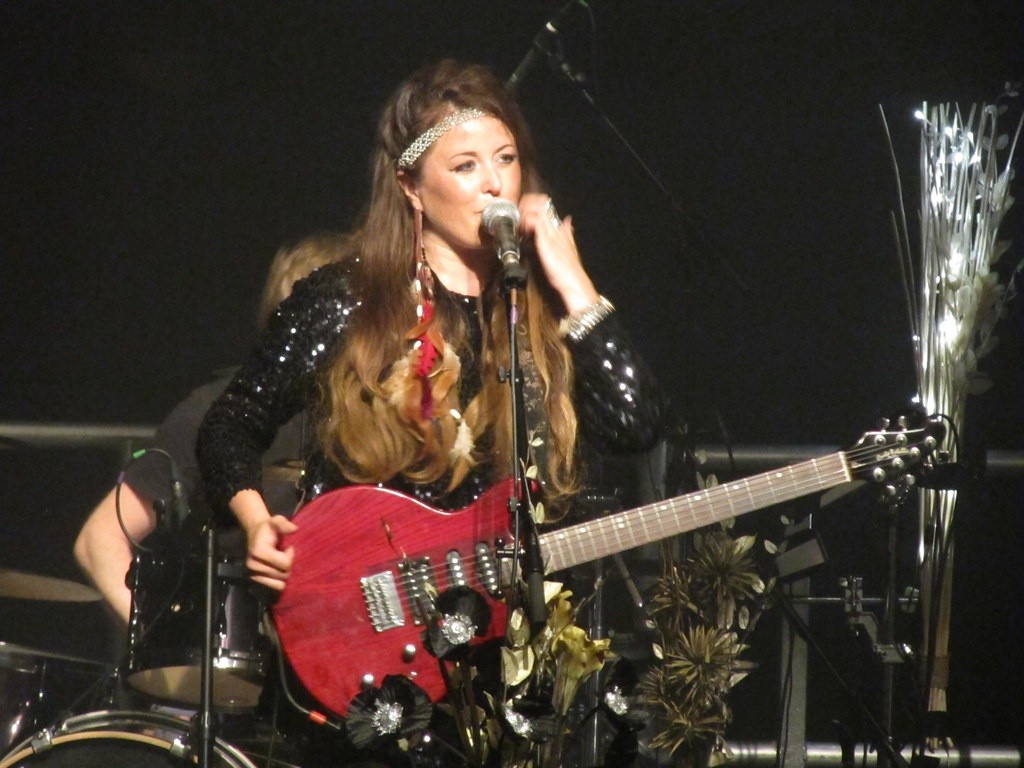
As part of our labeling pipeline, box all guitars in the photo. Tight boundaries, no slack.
[257,415,948,733]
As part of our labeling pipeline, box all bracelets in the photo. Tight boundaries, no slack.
[566,294,617,344]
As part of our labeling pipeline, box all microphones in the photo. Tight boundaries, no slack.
[481,199,527,287]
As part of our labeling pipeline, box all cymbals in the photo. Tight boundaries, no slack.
[0,567,105,604]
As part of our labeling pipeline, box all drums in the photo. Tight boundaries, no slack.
[0,641,114,765]
[0,664,391,768]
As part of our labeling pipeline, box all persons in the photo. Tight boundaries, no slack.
[73,230,360,710]
[191,58,671,768]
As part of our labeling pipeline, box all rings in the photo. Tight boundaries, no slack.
[545,197,563,229]
